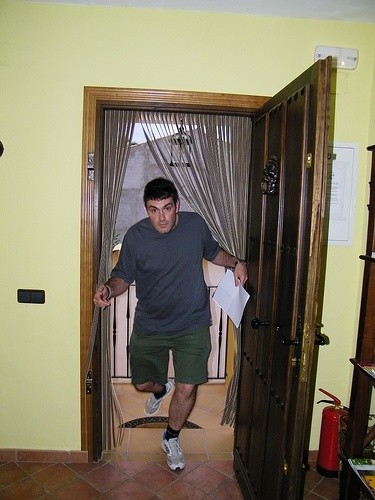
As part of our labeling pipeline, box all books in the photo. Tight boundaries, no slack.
[348,458,375,500]
[357,362,375,379]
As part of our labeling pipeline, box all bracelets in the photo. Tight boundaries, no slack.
[105,284,112,300]
[235,260,245,267]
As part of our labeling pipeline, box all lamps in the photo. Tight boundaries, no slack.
[169,118,193,168]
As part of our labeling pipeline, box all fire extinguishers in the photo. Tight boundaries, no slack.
[315,385,351,478]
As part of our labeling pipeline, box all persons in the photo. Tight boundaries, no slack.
[92,177,249,471]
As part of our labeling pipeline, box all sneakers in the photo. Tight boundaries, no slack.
[161,430,185,471]
[144,380,176,416]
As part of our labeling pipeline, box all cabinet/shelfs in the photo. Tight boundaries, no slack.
[341,144,375,500]
